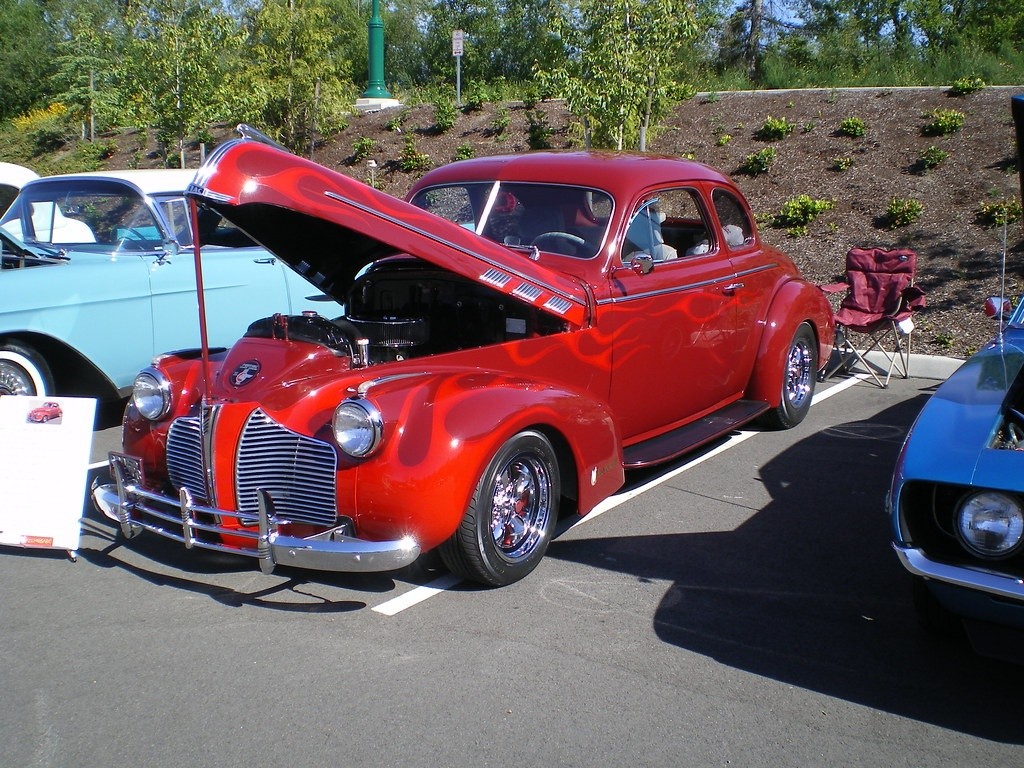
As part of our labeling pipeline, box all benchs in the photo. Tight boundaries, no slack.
[566,226,706,258]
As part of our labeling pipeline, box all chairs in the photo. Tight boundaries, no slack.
[149,207,222,247]
[519,203,578,257]
[817,248,927,389]
[621,199,677,267]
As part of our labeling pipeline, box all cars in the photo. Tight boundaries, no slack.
[883,292,1024,645]
[0,160,98,245]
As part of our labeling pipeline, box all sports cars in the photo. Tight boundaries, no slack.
[0,169,339,431]
[90,123,835,590]
